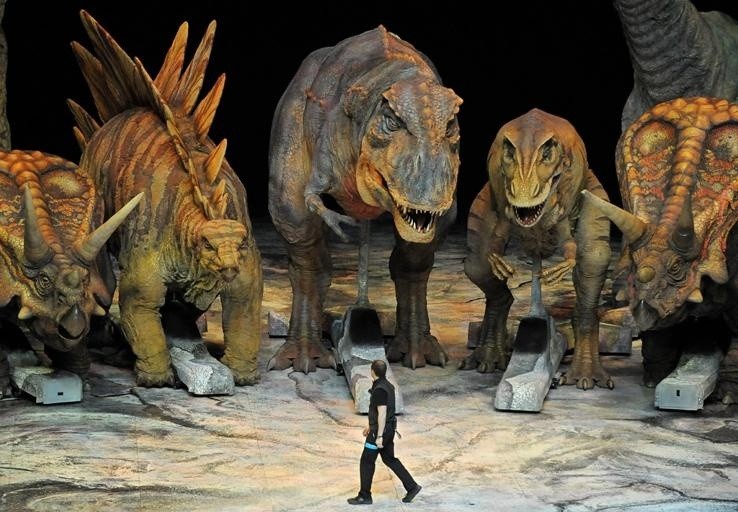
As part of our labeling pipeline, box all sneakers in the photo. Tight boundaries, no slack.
[402,483,422,503]
[347,492,373,505]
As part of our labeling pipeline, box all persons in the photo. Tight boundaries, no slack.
[345,359,424,506]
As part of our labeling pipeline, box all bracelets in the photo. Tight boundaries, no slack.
[375,434,383,439]
